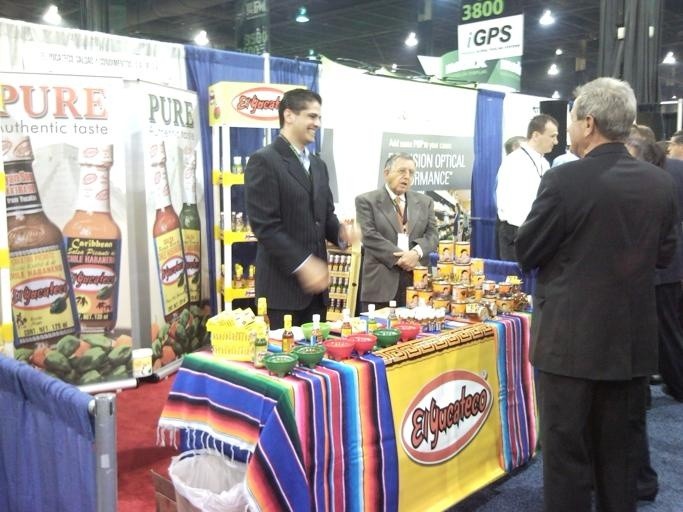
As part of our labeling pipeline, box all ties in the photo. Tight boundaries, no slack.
[396,196,405,231]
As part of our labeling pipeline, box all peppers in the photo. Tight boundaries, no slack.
[13,333,132,387]
[152,304,214,373]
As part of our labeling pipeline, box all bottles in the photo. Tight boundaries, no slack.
[1,133,81,351]
[396,305,446,334]
[252,297,399,368]
[62,137,122,333]
[325,253,351,315]
[176,147,202,308]
[219,154,255,292]
[141,138,190,323]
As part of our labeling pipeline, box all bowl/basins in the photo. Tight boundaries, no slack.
[262,323,421,378]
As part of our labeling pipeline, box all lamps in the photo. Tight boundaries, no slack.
[295,6,310,23]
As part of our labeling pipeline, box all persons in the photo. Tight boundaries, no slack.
[495,116,682,501]
[513,77,678,511]
[244,90,359,332]
[355,154,440,318]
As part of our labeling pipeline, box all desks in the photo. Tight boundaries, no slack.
[180,303,539,512]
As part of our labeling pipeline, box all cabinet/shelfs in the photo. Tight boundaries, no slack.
[326,246,360,321]
[208,81,308,314]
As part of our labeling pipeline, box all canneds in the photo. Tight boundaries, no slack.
[406,240,515,323]
